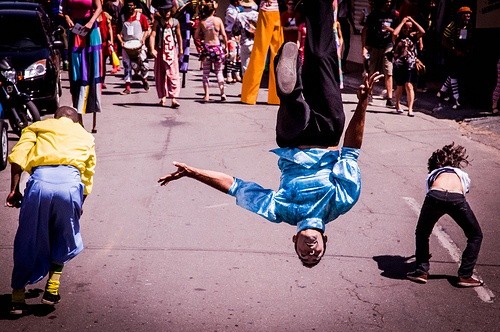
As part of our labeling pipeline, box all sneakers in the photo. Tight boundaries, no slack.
[406,269,428,282]
[457,276,483,287]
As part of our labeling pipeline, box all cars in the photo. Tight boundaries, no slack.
[0,1,67,114]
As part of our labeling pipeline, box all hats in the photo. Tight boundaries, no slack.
[457,6,473,13]
[159,0,172,8]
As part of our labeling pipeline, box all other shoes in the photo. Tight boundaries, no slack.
[408,112,414,116]
[41,290,61,304]
[276,41,299,94]
[368,97,373,104]
[386,101,396,107]
[9,302,24,314]
[397,108,404,113]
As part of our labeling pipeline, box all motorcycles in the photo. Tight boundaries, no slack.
[0,53,42,171]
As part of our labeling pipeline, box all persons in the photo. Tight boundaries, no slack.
[158,0,385,269]
[333,0,500,117]
[44,0,306,132]
[407,141,484,287]
[5,106,96,314]
